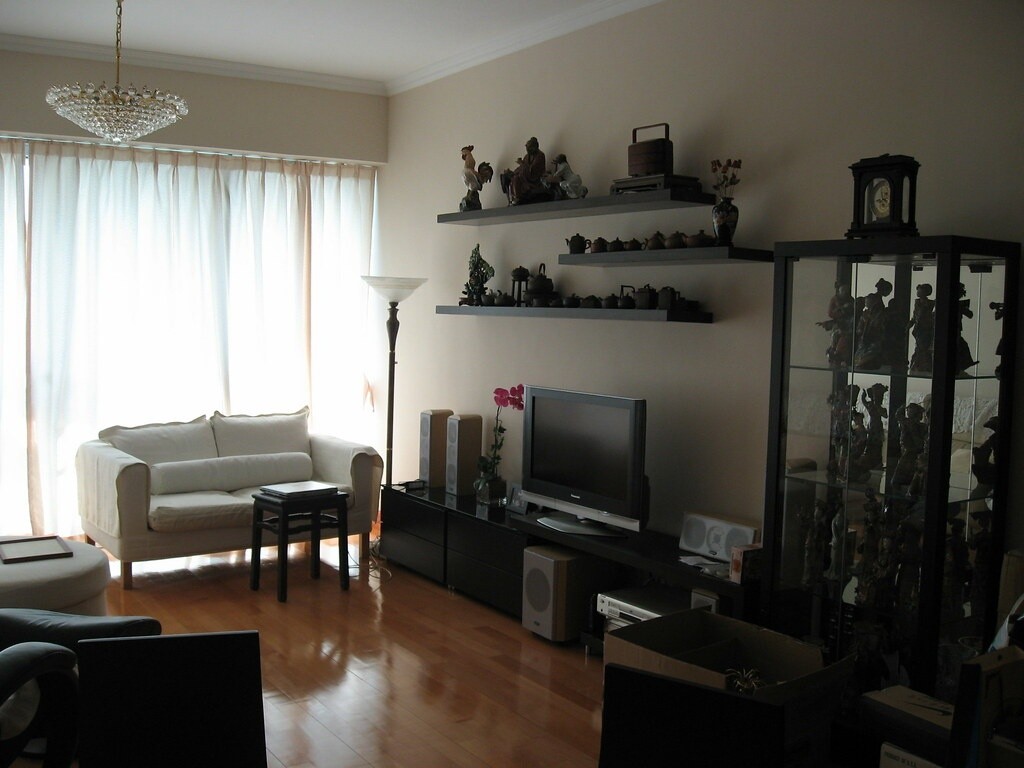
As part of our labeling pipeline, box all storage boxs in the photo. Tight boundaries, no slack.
[602,604,841,703]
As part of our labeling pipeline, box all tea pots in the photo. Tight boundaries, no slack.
[565,229,715,255]
[480,264,702,312]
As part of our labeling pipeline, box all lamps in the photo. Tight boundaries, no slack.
[363,275,429,553]
[46,0,189,145]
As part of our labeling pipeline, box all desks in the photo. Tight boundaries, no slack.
[252,492,350,602]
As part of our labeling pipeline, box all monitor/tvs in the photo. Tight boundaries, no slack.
[521,384,650,537]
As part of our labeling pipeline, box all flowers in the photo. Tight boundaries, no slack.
[481,385,524,475]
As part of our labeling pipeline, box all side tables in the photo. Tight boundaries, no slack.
[0,536,111,616]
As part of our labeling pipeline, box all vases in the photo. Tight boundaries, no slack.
[477,479,505,504]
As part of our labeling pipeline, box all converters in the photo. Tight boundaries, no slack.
[405,481,424,490]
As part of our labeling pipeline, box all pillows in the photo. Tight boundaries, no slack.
[98,415,218,468]
[150,451,313,495]
[210,405,310,456]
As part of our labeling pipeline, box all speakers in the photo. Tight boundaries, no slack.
[521,547,611,641]
[418,409,454,489]
[445,414,483,495]
[679,511,761,562]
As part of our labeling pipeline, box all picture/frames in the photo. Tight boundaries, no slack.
[0,535,73,564]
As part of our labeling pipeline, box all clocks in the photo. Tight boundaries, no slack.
[844,153,920,238]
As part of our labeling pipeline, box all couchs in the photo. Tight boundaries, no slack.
[76,434,384,588]
[0,606,161,768]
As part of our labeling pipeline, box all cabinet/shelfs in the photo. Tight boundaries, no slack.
[377,484,522,617]
[437,190,800,325]
[757,236,1024,768]
